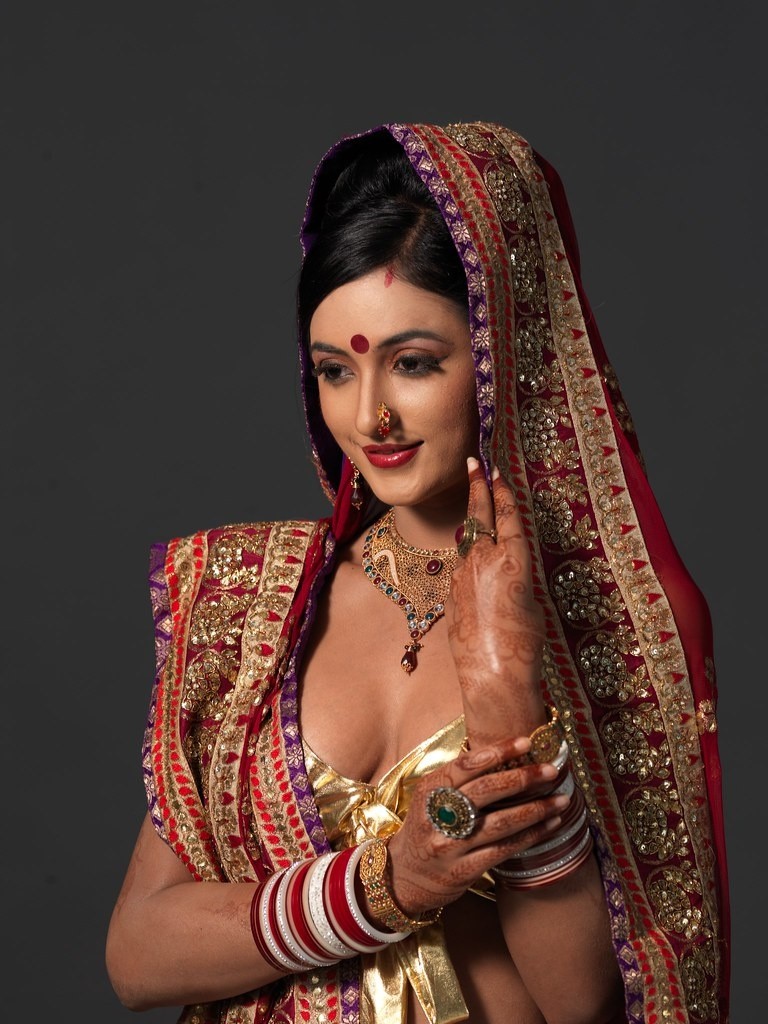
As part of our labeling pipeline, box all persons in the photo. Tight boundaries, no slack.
[105,121,732,1024]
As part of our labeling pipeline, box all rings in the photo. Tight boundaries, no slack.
[455,516,497,558]
[425,787,477,840]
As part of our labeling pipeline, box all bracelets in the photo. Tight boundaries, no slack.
[486,744,595,891]
[360,832,443,933]
[249,839,412,973]
[460,702,566,774]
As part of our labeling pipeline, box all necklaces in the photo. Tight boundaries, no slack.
[362,508,459,672]
